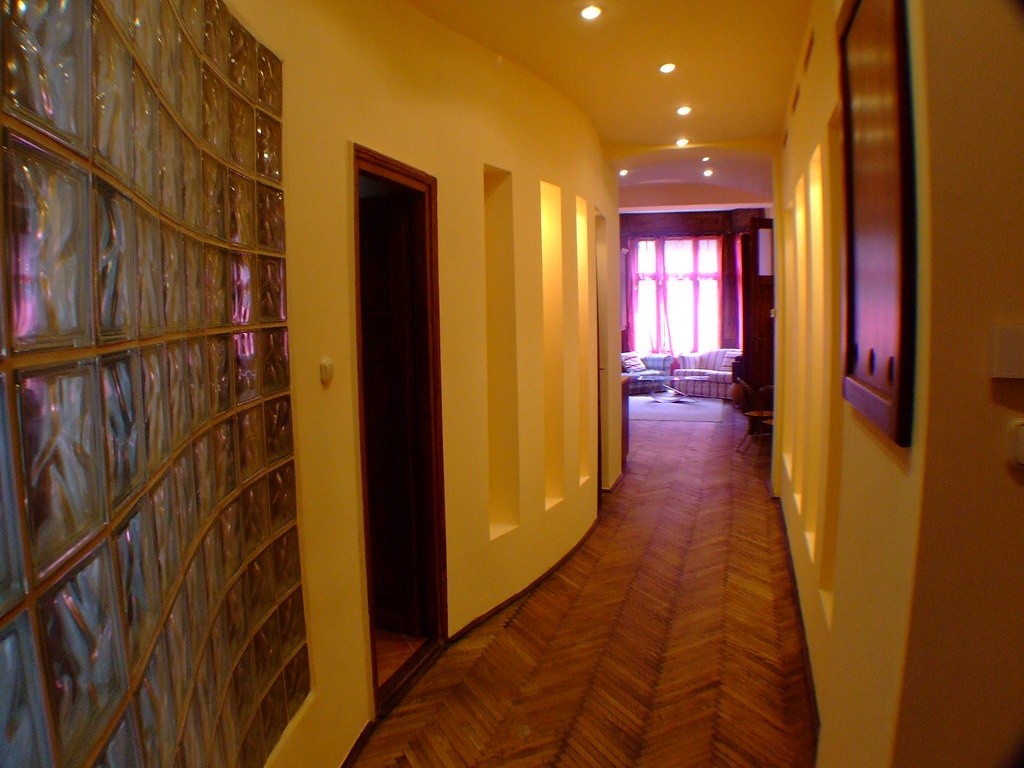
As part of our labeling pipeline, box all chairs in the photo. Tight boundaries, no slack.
[737,385,774,456]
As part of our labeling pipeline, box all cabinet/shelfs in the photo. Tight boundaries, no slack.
[732,218,776,412]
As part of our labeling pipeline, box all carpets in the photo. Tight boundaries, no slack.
[628,396,723,423]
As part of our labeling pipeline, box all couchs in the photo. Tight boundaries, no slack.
[621,350,673,395]
[674,349,743,398]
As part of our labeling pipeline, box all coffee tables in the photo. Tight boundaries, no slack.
[636,375,709,403]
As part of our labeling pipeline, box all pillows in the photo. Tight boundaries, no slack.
[621,351,646,373]
[721,352,743,371]
